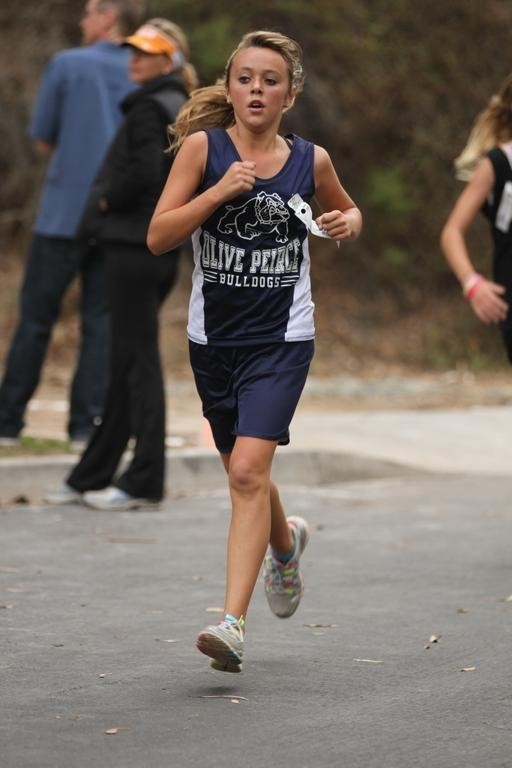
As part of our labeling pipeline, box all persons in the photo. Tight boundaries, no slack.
[0,1,144,454]
[41,17,199,512]
[146,30,364,672]
[440,74,512,370]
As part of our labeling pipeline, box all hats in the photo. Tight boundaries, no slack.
[119,25,183,67]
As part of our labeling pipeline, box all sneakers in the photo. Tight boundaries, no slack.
[264,516,310,618]
[195,613,245,672]
[43,483,160,511]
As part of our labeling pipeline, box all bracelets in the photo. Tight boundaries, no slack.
[462,273,484,301]
